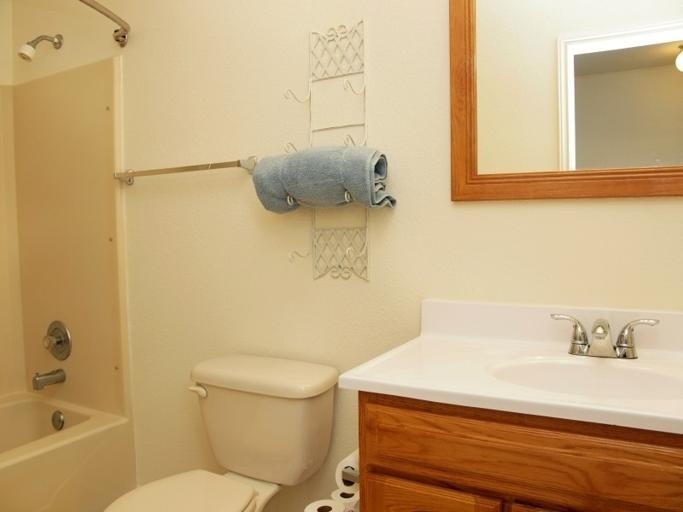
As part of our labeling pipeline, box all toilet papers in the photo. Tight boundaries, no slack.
[303,447,360,512]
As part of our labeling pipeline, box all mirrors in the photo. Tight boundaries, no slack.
[449,0,683,201]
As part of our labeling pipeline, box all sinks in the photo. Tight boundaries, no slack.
[494,352,683,414]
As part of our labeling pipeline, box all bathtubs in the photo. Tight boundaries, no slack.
[0,390,139,511]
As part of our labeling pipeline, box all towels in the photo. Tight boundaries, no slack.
[251,145,396,213]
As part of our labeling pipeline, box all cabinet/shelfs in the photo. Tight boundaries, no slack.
[359,391,683,512]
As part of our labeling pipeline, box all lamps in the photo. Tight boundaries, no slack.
[675,45,683,73]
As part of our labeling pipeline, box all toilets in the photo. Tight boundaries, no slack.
[108,356,340,511]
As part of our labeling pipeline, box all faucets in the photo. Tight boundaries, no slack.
[32,367,66,391]
[587,317,618,359]
